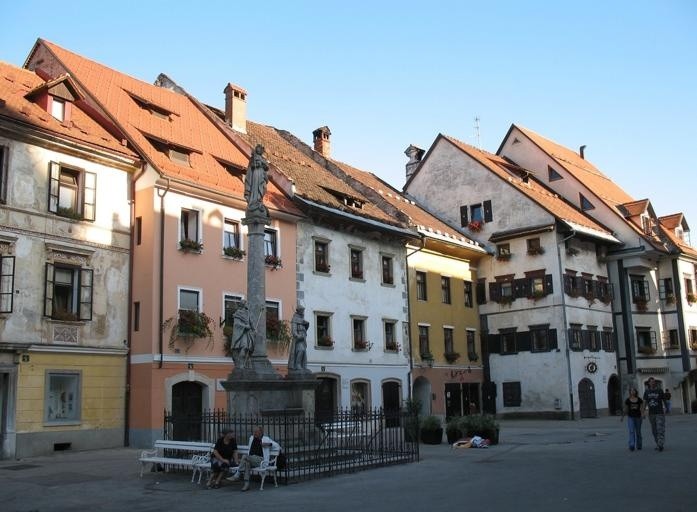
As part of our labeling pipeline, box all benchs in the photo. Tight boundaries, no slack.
[138,439,282,491]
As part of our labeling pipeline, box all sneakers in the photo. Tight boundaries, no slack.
[225,475,241,483]
[240,485,251,492]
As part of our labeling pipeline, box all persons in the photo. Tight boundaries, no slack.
[450,435,490,449]
[286,305,310,370]
[242,144,269,210]
[662,388,672,416]
[619,385,645,453]
[225,426,281,491]
[230,299,258,370]
[639,376,669,453]
[204,427,239,490]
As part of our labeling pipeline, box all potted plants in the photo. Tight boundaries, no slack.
[400,400,499,445]
